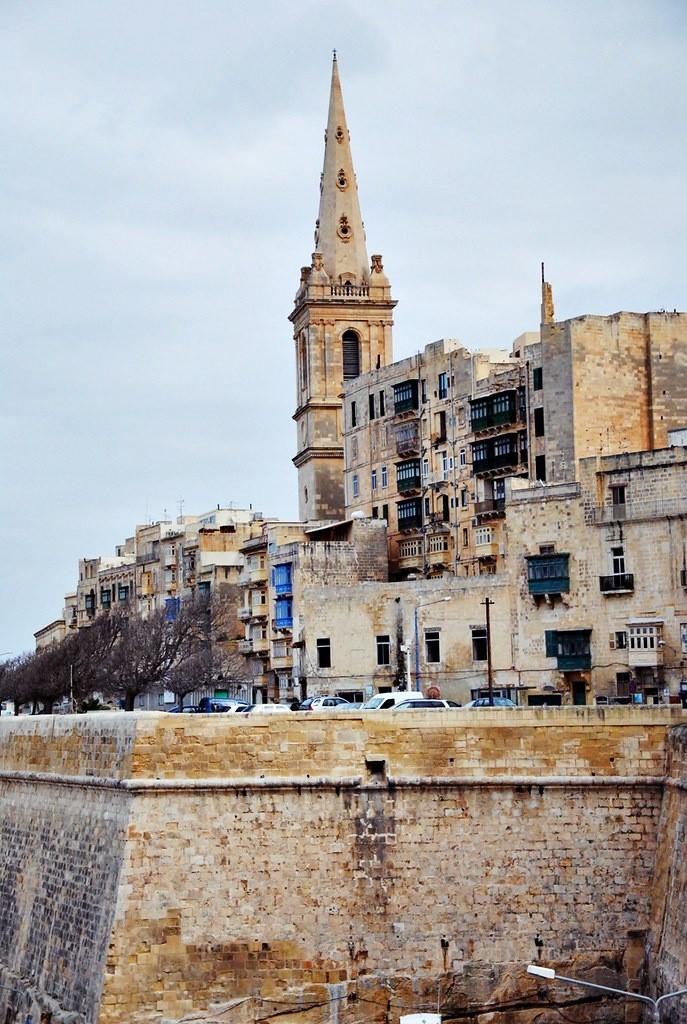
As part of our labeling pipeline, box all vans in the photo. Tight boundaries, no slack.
[198,697,249,712]
[363,693,423,710]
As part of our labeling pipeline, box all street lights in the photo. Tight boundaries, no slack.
[414,596,452,692]
[528,965,687,1024]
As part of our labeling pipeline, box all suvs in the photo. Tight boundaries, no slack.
[300,697,349,709]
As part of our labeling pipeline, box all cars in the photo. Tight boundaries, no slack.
[169,705,199,713]
[333,702,365,710]
[281,697,301,709]
[215,705,250,713]
[389,698,460,708]
[242,704,293,713]
[461,698,517,706]
